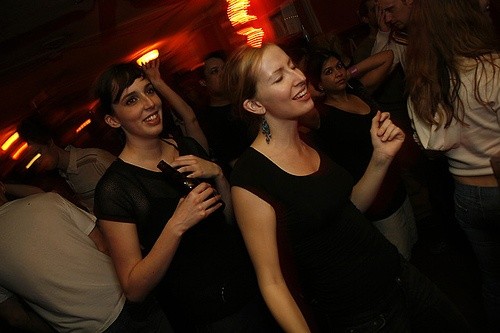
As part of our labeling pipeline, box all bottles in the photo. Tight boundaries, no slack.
[156,160,226,217]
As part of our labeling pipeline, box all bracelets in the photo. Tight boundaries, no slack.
[348,67,360,77]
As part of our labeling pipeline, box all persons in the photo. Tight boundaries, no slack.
[137,0,500,252]
[305,47,424,274]
[407,0,500,333]
[0,184,173,333]
[18,131,117,214]
[94,61,285,333]
[220,38,469,333]
